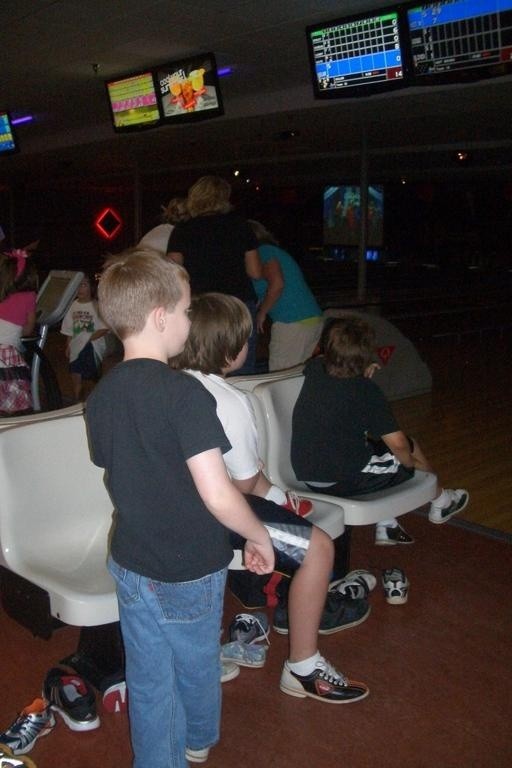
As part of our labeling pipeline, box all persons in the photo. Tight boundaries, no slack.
[290,316,472,545]
[167,173,263,377]
[82,253,275,767]
[246,220,327,372]
[133,196,190,253]
[65,271,116,404]
[170,294,370,705]
[1,248,41,416]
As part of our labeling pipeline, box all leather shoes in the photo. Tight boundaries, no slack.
[279,488,314,518]
[279,658,370,703]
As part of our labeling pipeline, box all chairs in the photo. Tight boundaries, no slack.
[226,360,306,391]
[1,396,88,431]
[1,414,120,689]
[227,389,345,571]
[252,374,438,526]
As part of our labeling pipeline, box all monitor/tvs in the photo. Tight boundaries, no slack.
[403,0,512,85]
[0,112,21,156]
[154,52,225,124]
[104,68,161,133]
[304,4,410,98]
[320,183,384,250]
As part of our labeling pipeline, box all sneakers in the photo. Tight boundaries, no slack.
[219,659,241,683]
[220,639,266,668]
[41,665,101,732]
[272,603,291,634]
[229,610,272,647]
[328,567,377,598]
[382,565,410,604]
[374,521,416,547]
[428,487,470,525]
[185,745,210,763]
[98,670,130,713]
[0,742,37,767]
[0,697,57,756]
[317,590,372,635]
